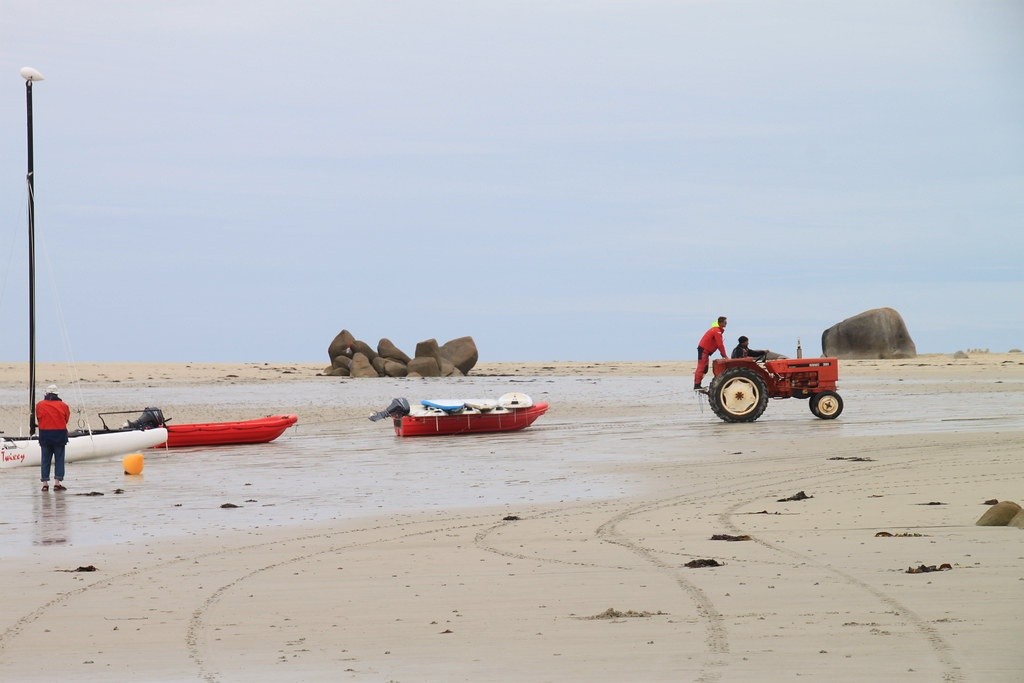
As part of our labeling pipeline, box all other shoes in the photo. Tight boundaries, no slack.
[694,385,700,389]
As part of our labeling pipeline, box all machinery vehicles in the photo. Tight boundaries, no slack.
[696,337,844,423]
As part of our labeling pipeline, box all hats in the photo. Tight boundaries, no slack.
[46,386,59,394]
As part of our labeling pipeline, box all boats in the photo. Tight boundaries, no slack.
[121,406,298,449]
[367,393,548,438]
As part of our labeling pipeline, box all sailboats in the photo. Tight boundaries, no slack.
[0,66,168,468]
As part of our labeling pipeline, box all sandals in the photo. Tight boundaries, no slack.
[42,486,48,491]
[54,485,67,490]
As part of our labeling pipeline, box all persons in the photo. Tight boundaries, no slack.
[35,384,71,491]
[731,336,769,359]
[693,316,729,389]
